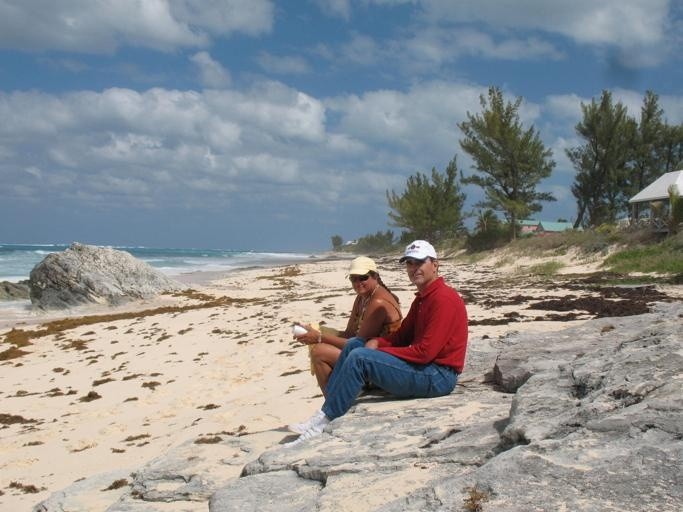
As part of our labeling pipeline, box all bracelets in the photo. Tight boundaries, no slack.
[317,330,321,343]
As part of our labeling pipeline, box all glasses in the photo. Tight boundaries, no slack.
[348,275,370,281]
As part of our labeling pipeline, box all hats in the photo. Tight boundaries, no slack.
[398,239,438,264]
[344,256,380,279]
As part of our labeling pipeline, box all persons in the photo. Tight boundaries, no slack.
[292,256,405,401]
[282,239,468,451]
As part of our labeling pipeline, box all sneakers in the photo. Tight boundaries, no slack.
[284,410,329,446]
